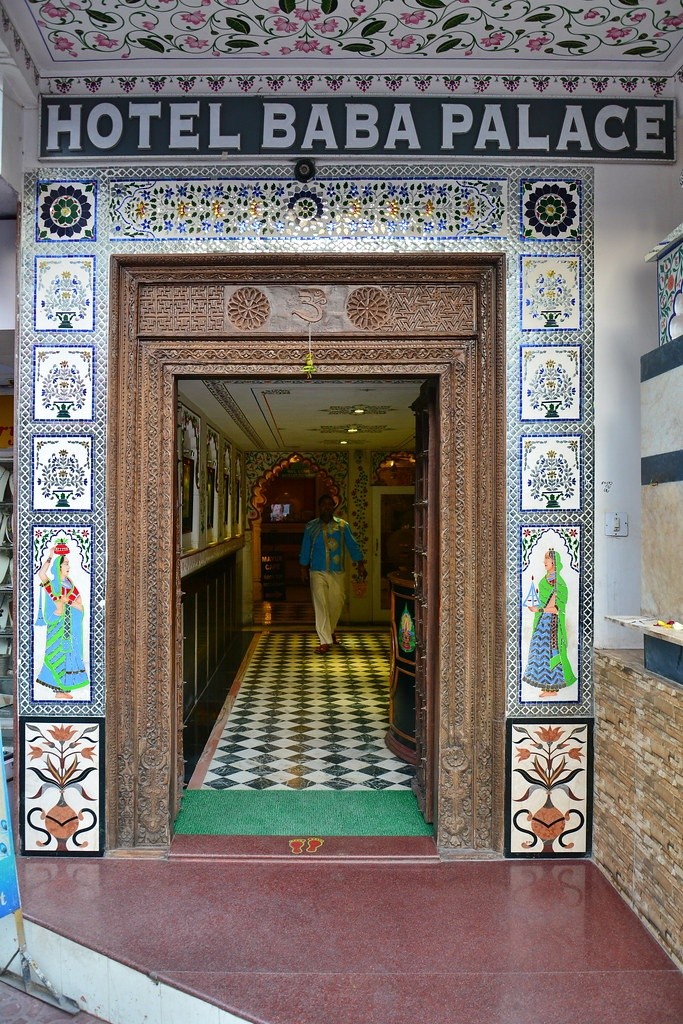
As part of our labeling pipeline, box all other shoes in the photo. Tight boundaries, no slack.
[331,633,339,644]
[314,644,330,654]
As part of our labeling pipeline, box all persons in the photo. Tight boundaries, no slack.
[298,495,367,655]
